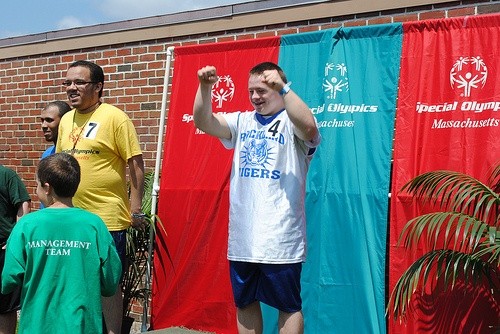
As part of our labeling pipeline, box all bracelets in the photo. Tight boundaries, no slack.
[279,82,292,95]
[131,212,144,218]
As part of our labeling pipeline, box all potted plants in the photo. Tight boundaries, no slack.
[102,170,168,334]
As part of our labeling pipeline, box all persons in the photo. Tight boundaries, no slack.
[0,164,32,334]
[192,61,321,334]
[39,101,71,209]
[0,153,123,334]
[55,61,145,334]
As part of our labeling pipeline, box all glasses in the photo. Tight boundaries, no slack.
[62,79,96,87]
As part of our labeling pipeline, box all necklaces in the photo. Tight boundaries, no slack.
[71,101,102,155]
